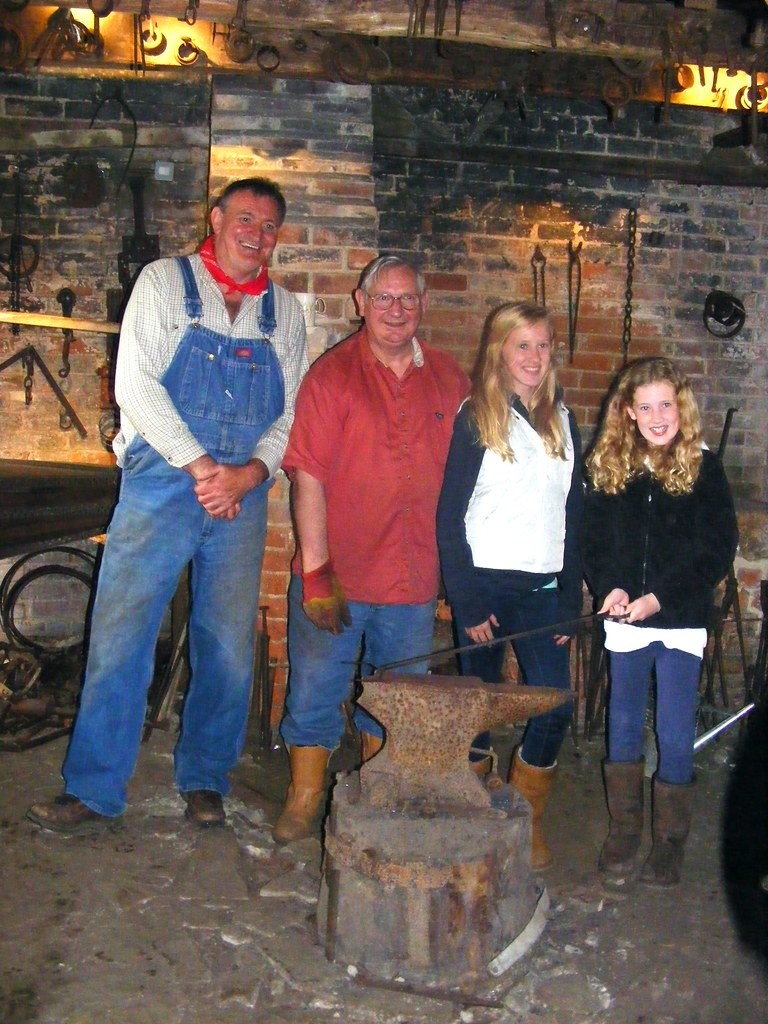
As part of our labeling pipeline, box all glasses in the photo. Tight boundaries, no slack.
[364,289,424,311]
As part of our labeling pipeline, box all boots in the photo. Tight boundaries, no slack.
[504,745,559,871]
[638,771,698,891]
[359,730,384,766]
[468,744,495,777]
[270,745,335,846]
[598,757,646,880]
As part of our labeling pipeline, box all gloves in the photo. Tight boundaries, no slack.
[433,593,455,621]
[302,559,352,635]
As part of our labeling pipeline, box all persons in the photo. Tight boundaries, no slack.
[271,255,476,846]
[27,178,308,831]
[584,357,740,887]
[434,301,586,870]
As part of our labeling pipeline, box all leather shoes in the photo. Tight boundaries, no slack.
[28,792,121,831]
[182,786,226,828]
